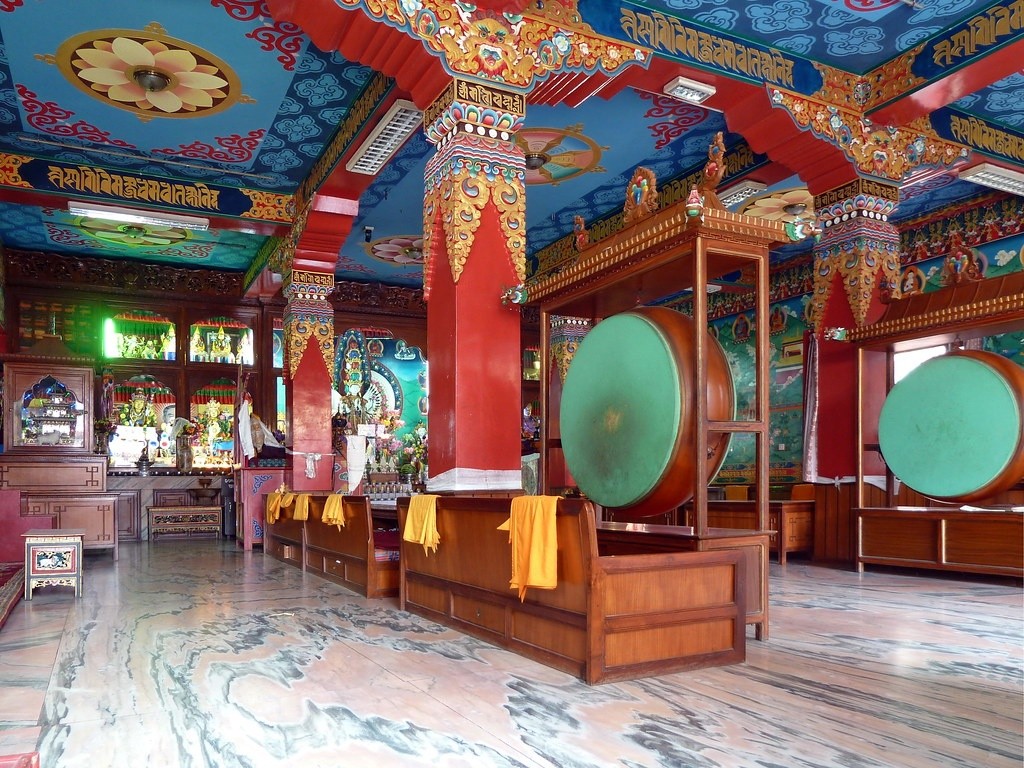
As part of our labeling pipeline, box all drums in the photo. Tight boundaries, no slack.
[877,349,1024,503]
[558,305,737,517]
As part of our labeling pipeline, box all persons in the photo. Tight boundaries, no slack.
[119,388,156,427]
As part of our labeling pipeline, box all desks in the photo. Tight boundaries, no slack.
[20,528,85,600]
[684,501,816,565]
[371,504,777,642]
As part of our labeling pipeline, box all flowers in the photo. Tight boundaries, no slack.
[176,420,204,440]
[370,410,406,455]
[404,445,429,465]
[93,418,117,437]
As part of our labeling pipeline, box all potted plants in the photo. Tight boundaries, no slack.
[401,463,416,484]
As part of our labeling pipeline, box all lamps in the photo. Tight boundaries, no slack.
[68,201,209,231]
[346,99,423,176]
[663,76,716,105]
[958,163,1024,196]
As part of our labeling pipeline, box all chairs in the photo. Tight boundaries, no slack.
[790,484,817,501]
[725,486,752,500]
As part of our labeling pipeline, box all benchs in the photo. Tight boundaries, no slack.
[262,493,746,686]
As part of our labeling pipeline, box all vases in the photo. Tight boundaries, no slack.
[177,435,193,476]
[423,465,428,484]
[93,433,111,474]
